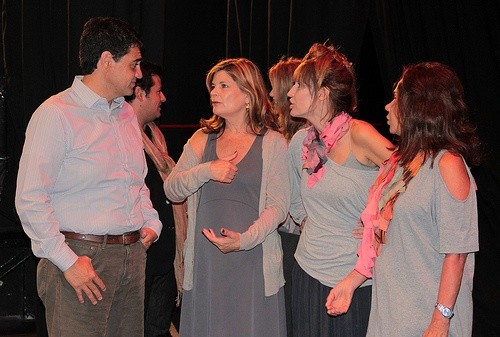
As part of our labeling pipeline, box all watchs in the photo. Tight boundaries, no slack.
[433,302,455,320]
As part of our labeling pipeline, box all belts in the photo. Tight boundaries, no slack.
[60,230,140,244]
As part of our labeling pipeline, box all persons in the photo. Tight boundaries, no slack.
[268,58,314,144]
[163,57,293,337]
[354,60,480,337]
[122,58,186,337]
[284,42,400,337]
[14,16,164,337]
